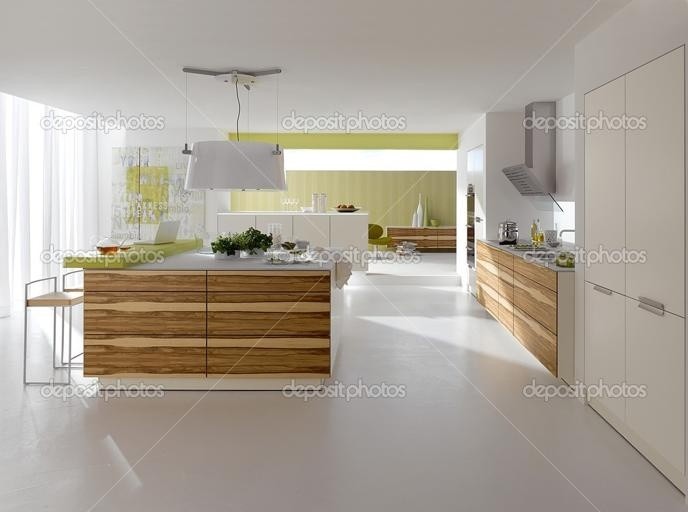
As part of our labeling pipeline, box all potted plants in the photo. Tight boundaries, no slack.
[212,232,242,258]
[237,227,273,259]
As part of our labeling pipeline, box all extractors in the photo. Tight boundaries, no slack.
[501,100,558,197]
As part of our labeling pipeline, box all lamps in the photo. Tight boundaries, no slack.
[181,140,287,192]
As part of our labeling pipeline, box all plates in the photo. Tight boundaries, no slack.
[330,207,362,213]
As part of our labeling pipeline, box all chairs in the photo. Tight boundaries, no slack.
[61,269,83,364]
[23,277,84,385]
[368,223,393,263]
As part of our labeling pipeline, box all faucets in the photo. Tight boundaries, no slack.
[559,229,575,237]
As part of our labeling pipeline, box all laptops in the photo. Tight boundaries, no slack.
[133,219,181,245]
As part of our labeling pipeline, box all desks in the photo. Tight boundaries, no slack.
[61,237,342,391]
[218,212,366,271]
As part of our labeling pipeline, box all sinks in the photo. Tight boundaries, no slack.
[527,253,559,259]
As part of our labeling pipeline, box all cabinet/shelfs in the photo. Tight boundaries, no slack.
[514,256,575,378]
[573,45,687,495]
[386,226,457,252]
[456,111,524,298]
[473,239,498,321]
[498,250,514,339]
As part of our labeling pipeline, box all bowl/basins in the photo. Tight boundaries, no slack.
[299,206,312,213]
[548,242,560,249]
[95,244,120,256]
